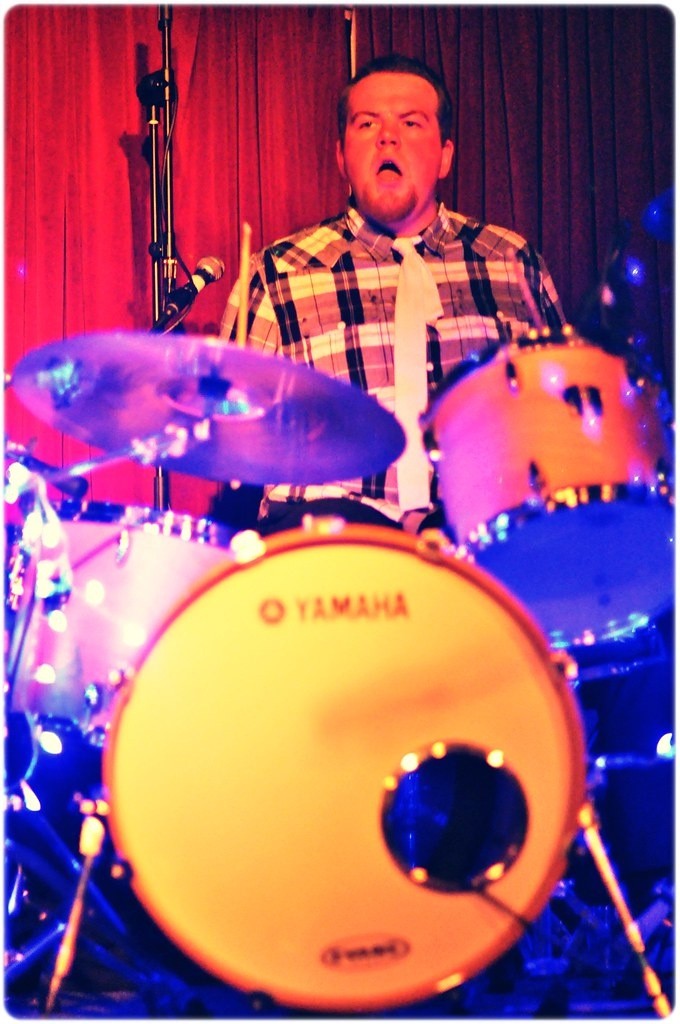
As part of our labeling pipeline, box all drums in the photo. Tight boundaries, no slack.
[4,320,675,1013]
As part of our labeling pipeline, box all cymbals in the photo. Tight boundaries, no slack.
[12,331,408,485]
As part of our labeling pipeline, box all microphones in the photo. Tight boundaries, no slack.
[151,254,224,333]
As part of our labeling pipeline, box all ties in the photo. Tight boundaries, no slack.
[391,236,444,512]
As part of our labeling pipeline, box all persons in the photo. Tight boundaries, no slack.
[220,59,577,545]
